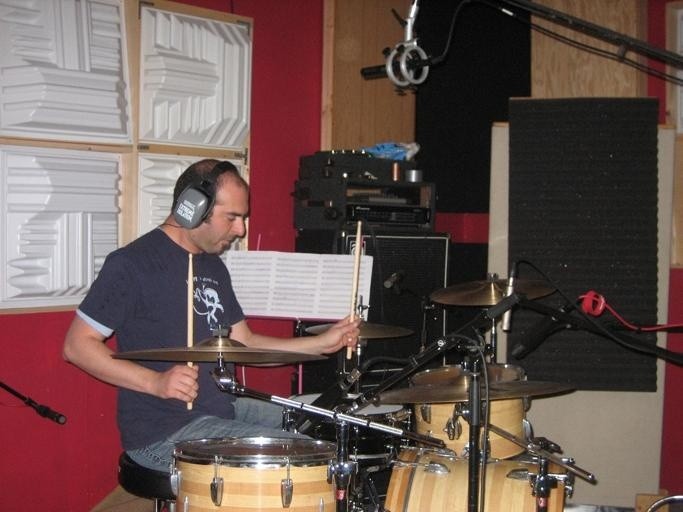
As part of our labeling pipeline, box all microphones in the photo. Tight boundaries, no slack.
[510,305,575,361]
[501,261,517,331]
[361,58,427,81]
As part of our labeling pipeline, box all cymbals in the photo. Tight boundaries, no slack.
[428,272,556,306]
[111,336,330,370]
[376,378,575,406]
[305,322,414,343]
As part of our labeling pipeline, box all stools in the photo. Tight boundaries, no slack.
[118,452,177,511]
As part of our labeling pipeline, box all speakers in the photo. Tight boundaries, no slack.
[296,220,449,512]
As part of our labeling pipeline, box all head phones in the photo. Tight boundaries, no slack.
[171,162,238,230]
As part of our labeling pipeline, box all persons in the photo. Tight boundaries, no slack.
[61,158,367,481]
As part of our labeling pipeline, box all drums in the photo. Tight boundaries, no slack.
[171,436,338,512]
[281,393,405,500]
[408,361,527,460]
[384,444,567,512]
